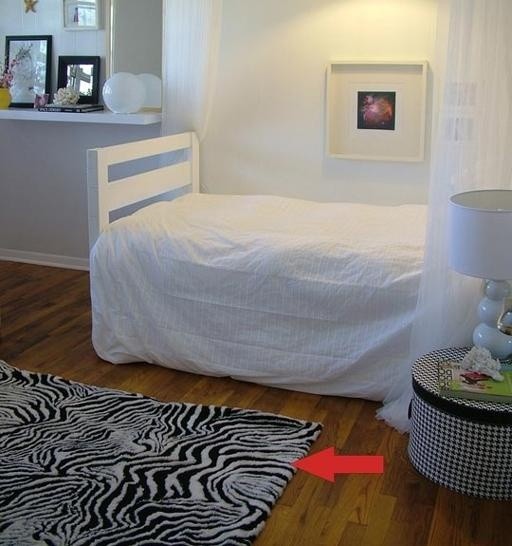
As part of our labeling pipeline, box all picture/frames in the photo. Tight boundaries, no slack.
[58,56,99,105]
[325,60,427,162]
[5,35,52,108]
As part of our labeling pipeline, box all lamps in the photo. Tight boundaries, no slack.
[446,188,512,362]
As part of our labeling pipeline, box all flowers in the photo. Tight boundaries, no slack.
[52,86,81,106]
[0,42,33,88]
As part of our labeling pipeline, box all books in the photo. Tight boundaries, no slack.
[437,361,512,402]
[39,104,104,112]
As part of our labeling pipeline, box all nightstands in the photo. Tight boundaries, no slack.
[406,344,512,503]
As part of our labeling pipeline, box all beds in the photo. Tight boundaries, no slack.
[86,130,429,403]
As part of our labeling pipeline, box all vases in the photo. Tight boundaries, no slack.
[0,87,12,109]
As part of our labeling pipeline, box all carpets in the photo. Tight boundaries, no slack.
[0,358,324,546]
